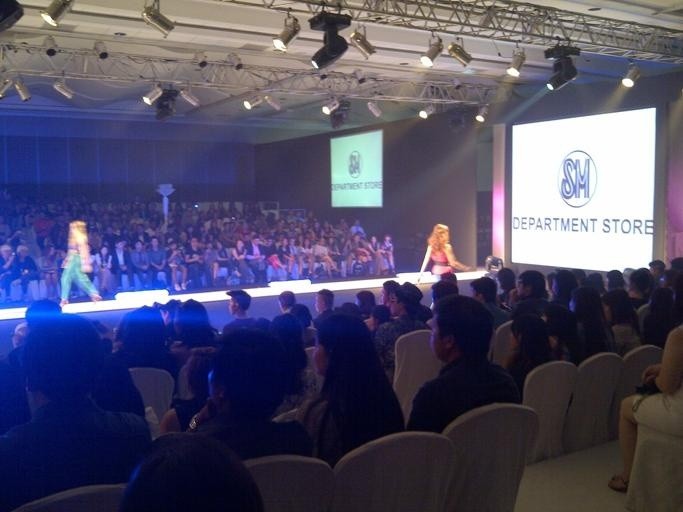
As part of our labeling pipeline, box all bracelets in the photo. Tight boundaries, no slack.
[188,414,201,432]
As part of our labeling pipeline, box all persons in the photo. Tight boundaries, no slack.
[167,198,395,291]
[562,288,629,362]
[508,271,553,362]
[642,287,678,345]
[588,273,604,290]
[469,278,512,323]
[0,315,152,512]
[60,192,100,217]
[169,300,218,346]
[432,278,460,304]
[59,220,103,307]
[602,283,642,351]
[483,253,503,277]
[117,410,263,511]
[277,292,297,313]
[313,290,335,330]
[87,235,102,290]
[115,299,176,378]
[608,269,626,287]
[671,257,683,270]
[506,314,559,390]
[629,271,659,301]
[380,281,399,305]
[406,294,516,428]
[540,303,577,357]
[373,284,429,367]
[0,187,57,302]
[312,313,405,465]
[388,286,398,304]
[103,197,164,292]
[608,324,683,497]
[220,290,257,334]
[290,304,321,347]
[363,306,391,330]
[354,291,374,318]
[1,299,63,374]
[649,257,667,278]
[549,270,577,299]
[497,268,516,305]
[659,270,679,293]
[419,223,458,278]
[184,329,314,456]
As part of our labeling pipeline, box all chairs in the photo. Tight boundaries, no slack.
[625,424,682,512]
[492,319,512,365]
[394,328,444,424]
[128,367,175,421]
[621,344,665,397]
[335,431,458,511]
[522,359,578,464]
[243,456,335,511]
[445,402,539,512]
[62,485,125,512]
[566,352,623,454]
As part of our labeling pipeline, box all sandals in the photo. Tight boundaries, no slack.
[608,475,630,493]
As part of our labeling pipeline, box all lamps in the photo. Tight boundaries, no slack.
[226,52,244,71]
[143,2,177,38]
[366,101,383,118]
[543,44,581,91]
[622,66,642,89]
[420,105,435,120]
[41,1,73,27]
[179,90,201,111]
[321,99,340,115]
[155,86,178,120]
[53,80,75,100]
[143,83,163,107]
[507,47,527,78]
[42,36,59,58]
[452,77,463,91]
[474,104,491,124]
[309,11,352,70]
[356,70,365,84]
[272,17,301,52]
[448,35,473,67]
[94,40,112,62]
[419,32,444,68]
[265,94,282,112]
[350,25,378,62]
[195,50,209,69]
[244,95,265,113]
[13,74,32,101]
